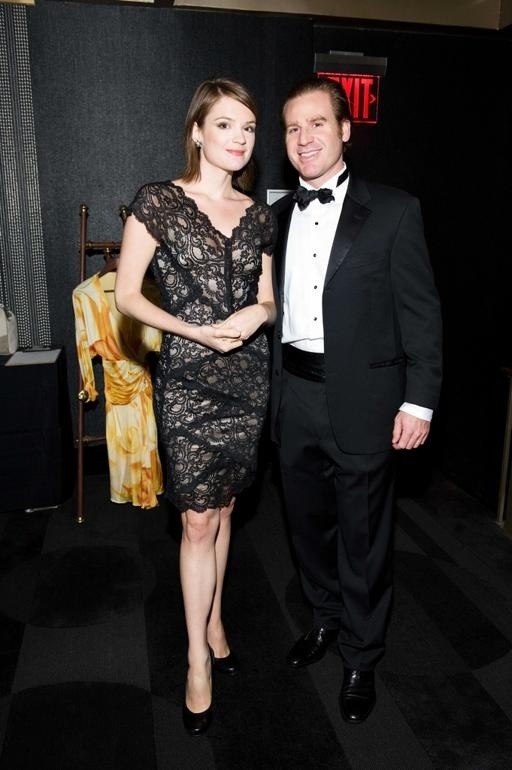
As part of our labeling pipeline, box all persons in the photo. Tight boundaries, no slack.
[267,75,446,724]
[113,75,278,737]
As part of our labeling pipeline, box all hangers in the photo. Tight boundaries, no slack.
[97,242,120,293]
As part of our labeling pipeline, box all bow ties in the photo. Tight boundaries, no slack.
[293,185,335,210]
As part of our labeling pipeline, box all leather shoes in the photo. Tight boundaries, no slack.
[286,623,334,670]
[183,644,237,735]
[339,668,376,725]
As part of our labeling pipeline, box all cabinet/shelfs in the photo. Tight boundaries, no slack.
[0,347,68,512]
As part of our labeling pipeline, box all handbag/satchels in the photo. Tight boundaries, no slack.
[0,303,19,356]
